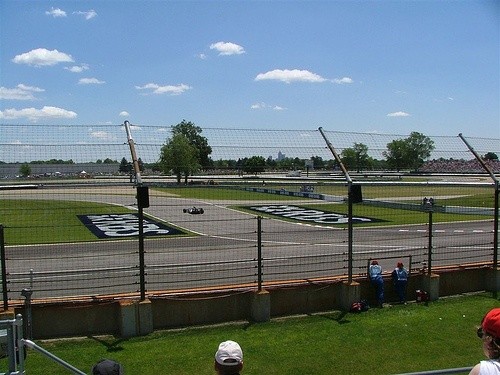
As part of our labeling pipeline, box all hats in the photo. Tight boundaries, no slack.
[397,261,404,267]
[371,261,378,265]
[215,340,243,365]
[481,308,500,338]
[91,359,121,375]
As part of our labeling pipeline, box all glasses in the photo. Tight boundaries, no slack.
[477,328,489,339]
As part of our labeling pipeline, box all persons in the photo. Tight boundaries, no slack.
[469,308,500,375]
[391,261,408,304]
[214,340,243,375]
[369,261,384,307]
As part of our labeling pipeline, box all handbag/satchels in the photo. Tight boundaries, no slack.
[351,298,369,312]
